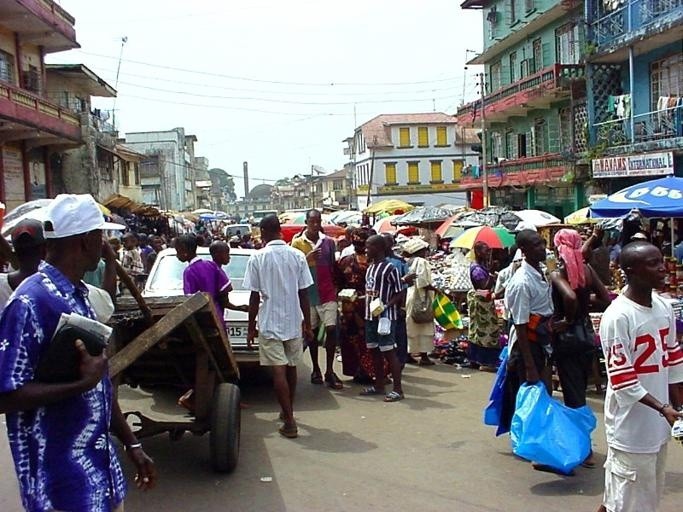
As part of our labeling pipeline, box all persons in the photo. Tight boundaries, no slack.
[611,88,629,144]
[191,176,680,252]
[493,156,510,170]
[0,191,156,512]
[531,226,612,476]
[1,205,683,439]
[597,241,682,512]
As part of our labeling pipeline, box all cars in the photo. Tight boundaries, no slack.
[140,246,277,378]
[218,206,367,246]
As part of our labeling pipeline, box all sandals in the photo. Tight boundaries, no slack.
[279,423,297,438]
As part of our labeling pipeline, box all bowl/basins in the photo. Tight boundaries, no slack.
[431,291,463,331]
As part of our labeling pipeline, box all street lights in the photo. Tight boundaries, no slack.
[112,34,132,141]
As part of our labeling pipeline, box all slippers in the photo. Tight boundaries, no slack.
[311,356,435,402]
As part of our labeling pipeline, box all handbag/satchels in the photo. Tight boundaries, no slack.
[31,324,106,406]
[411,288,434,323]
[527,316,553,345]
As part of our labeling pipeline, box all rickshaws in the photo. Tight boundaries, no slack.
[80,241,242,477]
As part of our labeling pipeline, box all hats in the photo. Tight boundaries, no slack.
[11,218,48,249]
[42,194,126,240]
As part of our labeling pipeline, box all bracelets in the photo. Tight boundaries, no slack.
[121,442,145,453]
[658,403,671,416]
